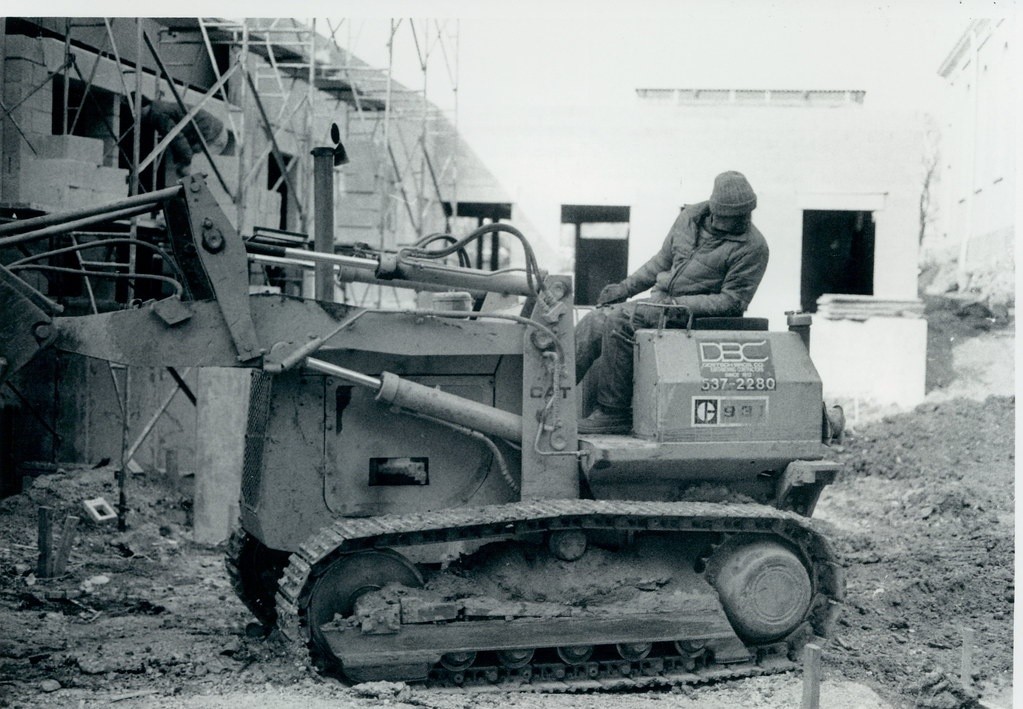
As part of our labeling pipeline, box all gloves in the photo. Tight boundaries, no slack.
[597,283,628,304]
[643,300,680,325]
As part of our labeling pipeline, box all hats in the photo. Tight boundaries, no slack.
[710,170,756,216]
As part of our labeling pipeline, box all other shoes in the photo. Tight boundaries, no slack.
[578,408,632,433]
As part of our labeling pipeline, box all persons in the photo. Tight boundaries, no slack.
[574,172,768,434]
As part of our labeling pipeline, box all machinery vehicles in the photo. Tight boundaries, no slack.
[2,172,845,693]
